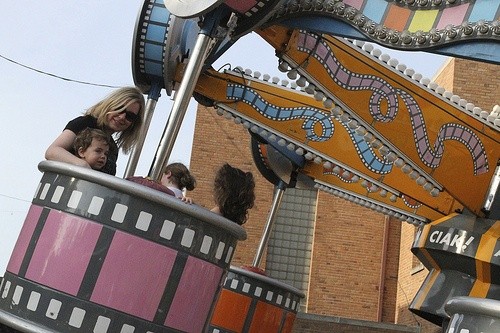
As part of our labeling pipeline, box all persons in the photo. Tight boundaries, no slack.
[73,126,111,173]
[161,163,196,205]
[176,162,256,226]
[44,85,148,177]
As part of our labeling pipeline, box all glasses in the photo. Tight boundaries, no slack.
[113,105,138,124]
